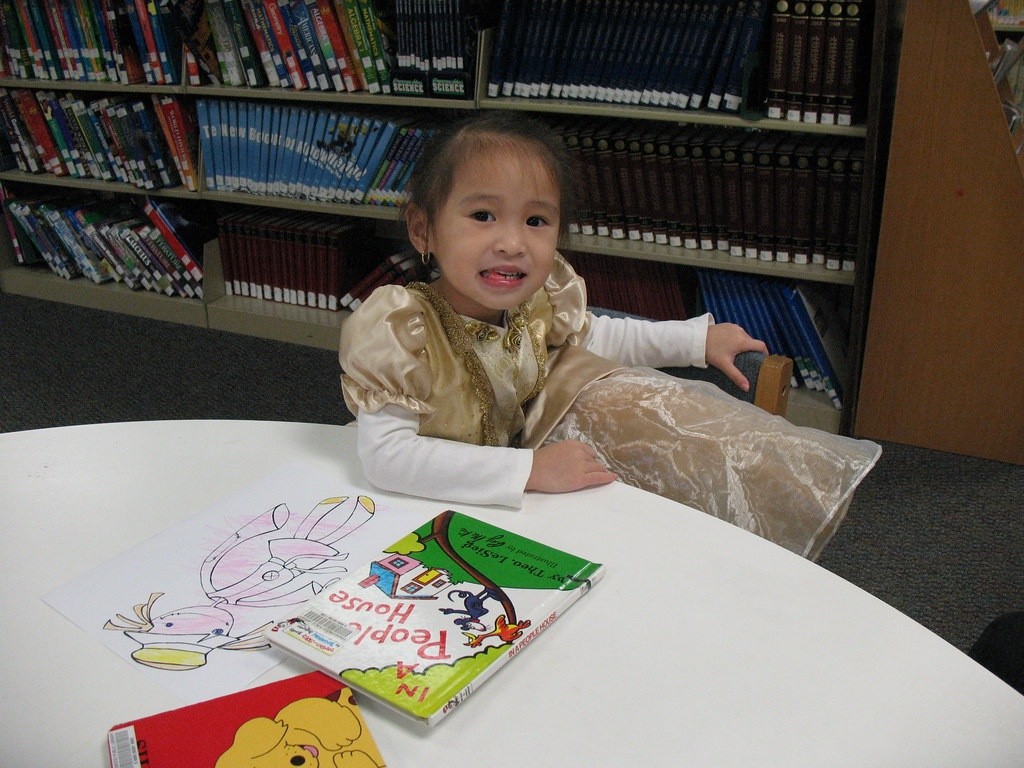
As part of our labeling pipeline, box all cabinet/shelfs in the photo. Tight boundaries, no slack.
[0,0,1024,435]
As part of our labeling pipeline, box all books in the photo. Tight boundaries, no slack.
[261,511,604,728]
[108,672,388,768]
[989,0,1024,104]
[0,1,874,411]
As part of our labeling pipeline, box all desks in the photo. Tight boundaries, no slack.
[0,421,1024,768]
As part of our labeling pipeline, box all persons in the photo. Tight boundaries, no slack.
[338,112,768,508]
